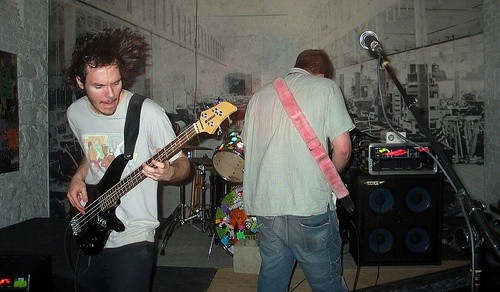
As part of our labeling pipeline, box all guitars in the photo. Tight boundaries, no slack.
[64,97,238,276]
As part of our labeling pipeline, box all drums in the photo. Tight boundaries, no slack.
[214,185,258,256]
[212,132,245,182]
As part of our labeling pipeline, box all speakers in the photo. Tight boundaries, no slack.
[348,170,444,267]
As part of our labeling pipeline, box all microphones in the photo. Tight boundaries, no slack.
[359,30,390,69]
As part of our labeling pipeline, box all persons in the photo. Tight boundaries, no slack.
[60,27,192,292]
[242,46,357,292]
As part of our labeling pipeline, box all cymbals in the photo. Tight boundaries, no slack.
[180,144,212,150]
[188,156,212,165]
